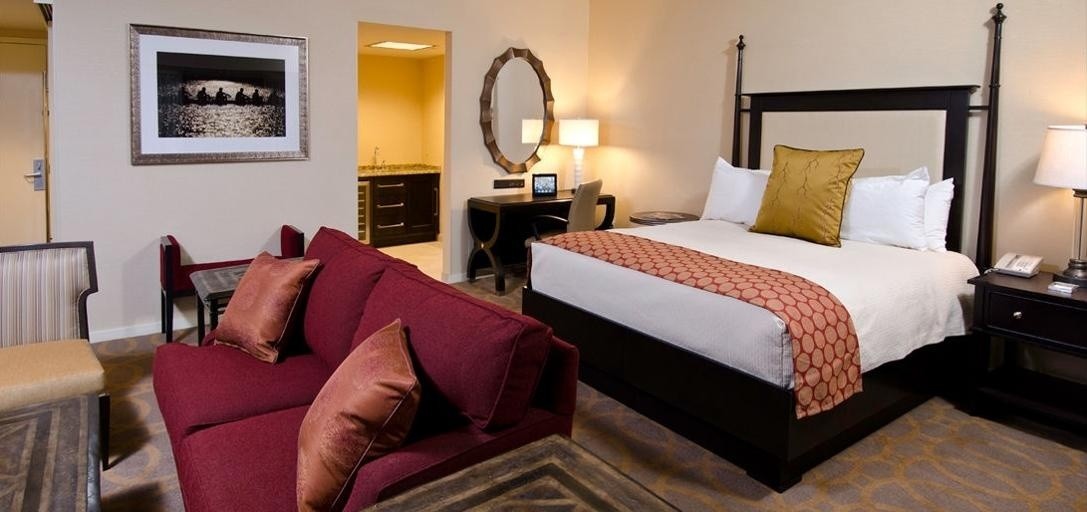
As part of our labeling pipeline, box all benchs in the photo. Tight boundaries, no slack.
[158,222,305,343]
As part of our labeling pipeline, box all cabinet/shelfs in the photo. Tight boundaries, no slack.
[370,173,407,249]
[358,175,371,247]
[406,171,442,244]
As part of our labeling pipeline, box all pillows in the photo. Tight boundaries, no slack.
[209,249,323,364]
[697,144,957,250]
[291,313,428,512]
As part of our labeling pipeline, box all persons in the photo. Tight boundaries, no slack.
[235,88,251,107]
[215,88,231,106]
[196,87,210,106]
[252,89,264,107]
[268,90,283,104]
[178,87,192,105]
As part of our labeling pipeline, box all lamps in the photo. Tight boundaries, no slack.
[1034,123,1087,287]
[557,116,601,193]
[519,118,544,145]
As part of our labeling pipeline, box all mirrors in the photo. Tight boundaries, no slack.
[478,46,556,177]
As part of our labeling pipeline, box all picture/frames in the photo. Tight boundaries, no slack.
[126,19,312,168]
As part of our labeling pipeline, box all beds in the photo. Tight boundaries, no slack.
[519,1,1007,494]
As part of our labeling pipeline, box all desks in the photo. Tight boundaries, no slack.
[627,210,700,224]
[189,263,250,347]
[464,188,616,298]
[1,390,104,512]
[357,424,681,512]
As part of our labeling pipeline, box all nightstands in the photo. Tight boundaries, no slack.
[964,261,1087,455]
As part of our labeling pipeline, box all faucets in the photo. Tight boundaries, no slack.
[373,145,380,168]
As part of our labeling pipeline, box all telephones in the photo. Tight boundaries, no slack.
[993,252,1044,278]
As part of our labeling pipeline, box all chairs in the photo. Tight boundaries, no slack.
[524,177,604,250]
[0,237,115,473]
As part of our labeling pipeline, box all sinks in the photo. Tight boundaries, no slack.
[364,165,400,173]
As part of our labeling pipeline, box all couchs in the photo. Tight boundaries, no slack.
[150,220,583,512]
